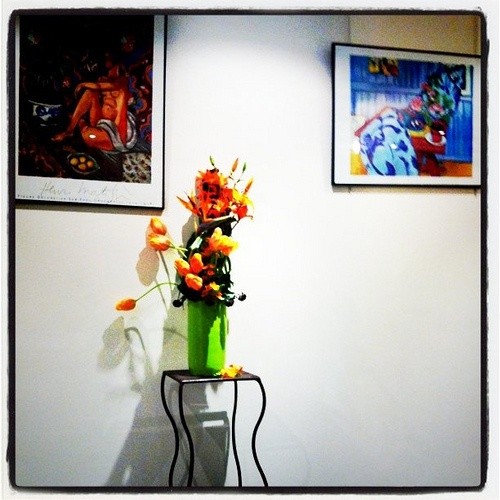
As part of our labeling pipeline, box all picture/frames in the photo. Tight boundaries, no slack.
[16,13,166,212]
[330,38,484,188]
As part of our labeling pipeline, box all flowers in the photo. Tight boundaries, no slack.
[113,157,256,311]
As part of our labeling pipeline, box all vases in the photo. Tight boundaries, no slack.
[185,298,229,375]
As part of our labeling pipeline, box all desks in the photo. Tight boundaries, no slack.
[159,368,274,486]
